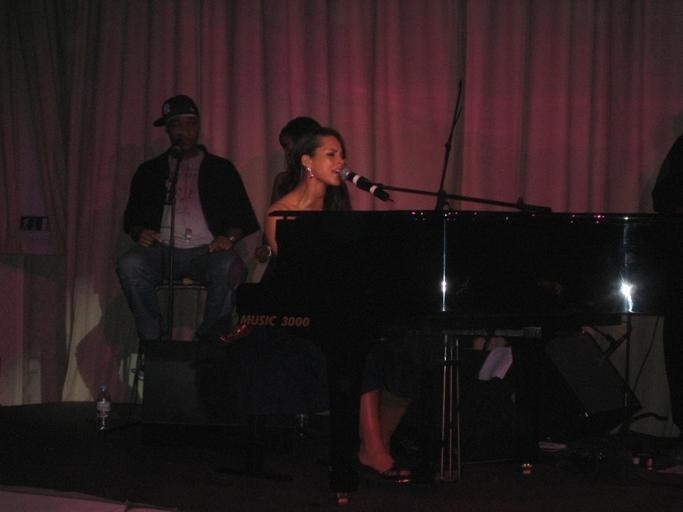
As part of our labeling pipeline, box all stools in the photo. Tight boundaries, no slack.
[124,277,210,423]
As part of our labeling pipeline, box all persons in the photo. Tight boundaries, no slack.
[265,127,415,487]
[651,136,683,429]
[115,95,260,344]
[270,115,353,210]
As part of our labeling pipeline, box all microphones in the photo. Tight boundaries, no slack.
[340,168,389,200]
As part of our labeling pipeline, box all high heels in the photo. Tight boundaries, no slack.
[356,453,417,487]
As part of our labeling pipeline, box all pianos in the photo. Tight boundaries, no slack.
[234,211,683,483]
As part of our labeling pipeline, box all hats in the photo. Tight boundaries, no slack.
[151,94,200,127]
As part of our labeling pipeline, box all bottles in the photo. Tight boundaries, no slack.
[95,385,111,431]
